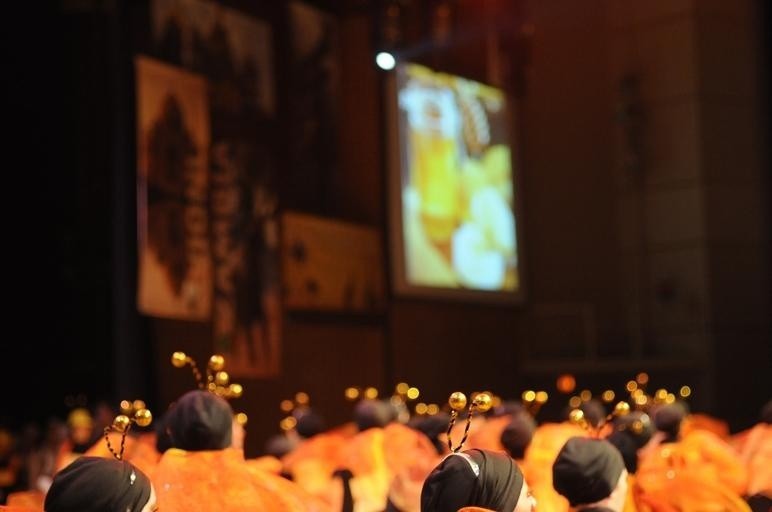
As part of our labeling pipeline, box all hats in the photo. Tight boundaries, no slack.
[419,450,524,512]
[45,456,151,512]
[553,434,625,506]
[168,390,233,449]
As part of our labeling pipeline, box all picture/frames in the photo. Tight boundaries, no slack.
[385,58,529,311]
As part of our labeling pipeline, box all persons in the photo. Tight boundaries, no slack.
[1,397,538,511]
[523,400,772,512]
[221,190,271,368]
